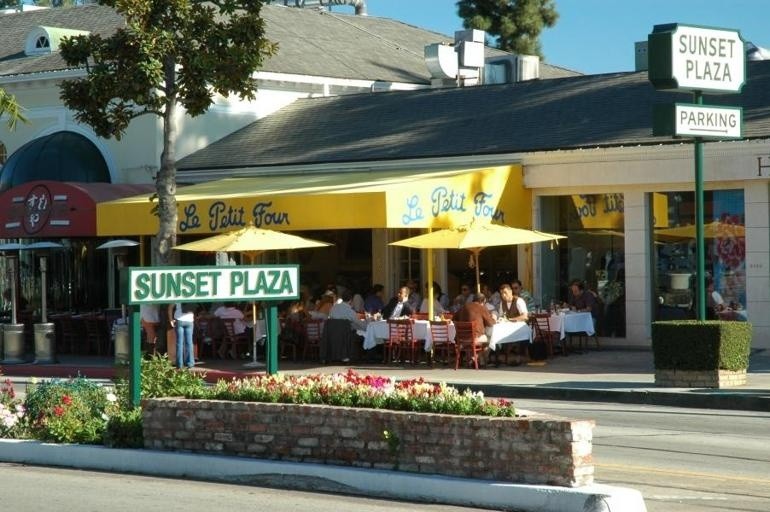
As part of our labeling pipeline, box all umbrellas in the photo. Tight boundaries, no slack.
[383,215,569,362]
[653,218,746,291]
[555,227,624,291]
[168,218,338,360]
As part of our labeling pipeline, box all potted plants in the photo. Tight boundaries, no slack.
[664,247,696,289]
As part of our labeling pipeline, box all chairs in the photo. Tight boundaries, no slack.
[91,288,603,373]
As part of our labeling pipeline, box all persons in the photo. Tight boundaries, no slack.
[277,284,375,367]
[563,281,599,314]
[363,279,450,367]
[184,301,267,366]
[452,280,536,368]
[140,304,161,359]
[579,274,747,337]
